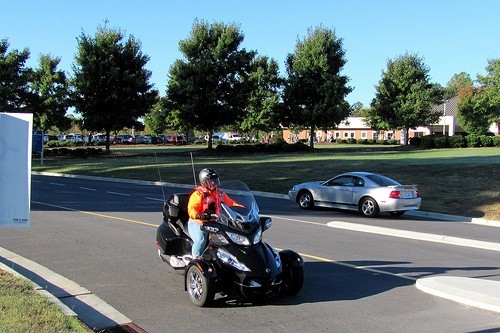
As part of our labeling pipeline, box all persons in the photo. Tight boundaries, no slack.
[187,168,245,262]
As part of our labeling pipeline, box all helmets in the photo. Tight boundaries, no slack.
[198,168,221,188]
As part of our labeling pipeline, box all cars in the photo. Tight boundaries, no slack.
[228,135,246,143]
[288,171,422,217]
[45,132,191,147]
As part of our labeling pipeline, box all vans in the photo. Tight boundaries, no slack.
[204,134,220,143]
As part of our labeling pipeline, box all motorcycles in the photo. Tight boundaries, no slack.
[154,180,306,307]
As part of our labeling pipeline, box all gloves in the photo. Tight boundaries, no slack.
[201,212,212,221]
